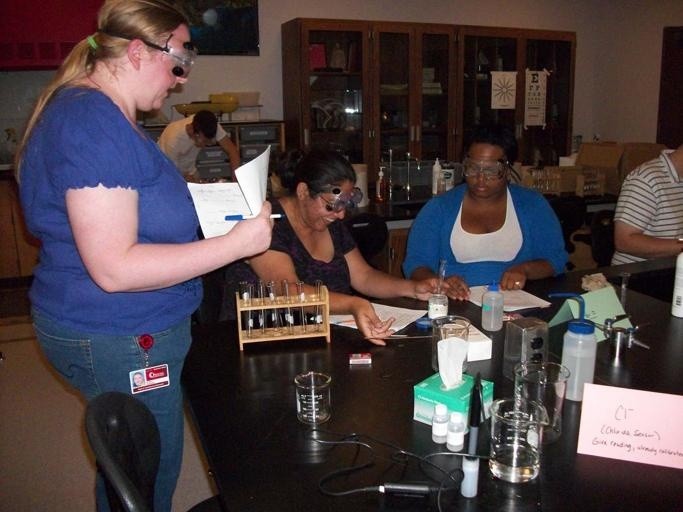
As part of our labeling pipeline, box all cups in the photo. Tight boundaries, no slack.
[350,164,370,208]
[430,315,471,373]
[293,369,332,426]
[525,164,601,195]
[489,397,550,484]
[428,293,449,320]
[512,357,572,444]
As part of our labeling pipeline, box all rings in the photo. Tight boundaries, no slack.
[515,281,520,286]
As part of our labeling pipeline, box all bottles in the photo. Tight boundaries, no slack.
[242,279,323,339]
[482,278,505,330]
[548,292,599,402]
[445,412,464,452]
[431,405,448,444]
[670,248,683,317]
[432,156,456,197]
[436,258,448,293]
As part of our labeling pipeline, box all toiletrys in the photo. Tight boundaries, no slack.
[437,171,446,195]
[672,238,683,318]
[376,166,387,202]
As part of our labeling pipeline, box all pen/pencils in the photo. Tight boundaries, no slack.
[224,213,285,221]
[336,319,355,325]
[678,238,683,241]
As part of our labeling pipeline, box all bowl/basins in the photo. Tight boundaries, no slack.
[225,91,262,106]
[174,101,239,114]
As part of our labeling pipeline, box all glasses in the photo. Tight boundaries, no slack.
[307,184,364,213]
[462,156,510,182]
[97,27,196,81]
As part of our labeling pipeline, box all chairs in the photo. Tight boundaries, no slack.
[343,210,387,270]
[587,208,617,270]
[82,388,219,512]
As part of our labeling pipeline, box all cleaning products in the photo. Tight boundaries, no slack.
[432,158,441,194]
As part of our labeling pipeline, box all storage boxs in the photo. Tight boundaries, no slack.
[138,117,286,184]
[579,141,666,196]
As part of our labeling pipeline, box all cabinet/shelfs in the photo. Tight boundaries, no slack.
[0,164,44,280]
[459,25,577,168]
[373,21,458,187]
[0,0,106,72]
[280,18,373,187]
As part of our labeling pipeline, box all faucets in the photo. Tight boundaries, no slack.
[402,152,421,192]
[593,315,650,367]
[380,149,393,193]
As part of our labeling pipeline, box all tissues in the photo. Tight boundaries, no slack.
[413,337,494,435]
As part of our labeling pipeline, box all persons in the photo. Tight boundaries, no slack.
[156,111,240,182]
[610,143,683,265]
[402,134,570,301]
[12,0,275,512]
[216,149,449,346]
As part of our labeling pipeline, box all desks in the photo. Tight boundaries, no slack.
[183,255,683,509]
